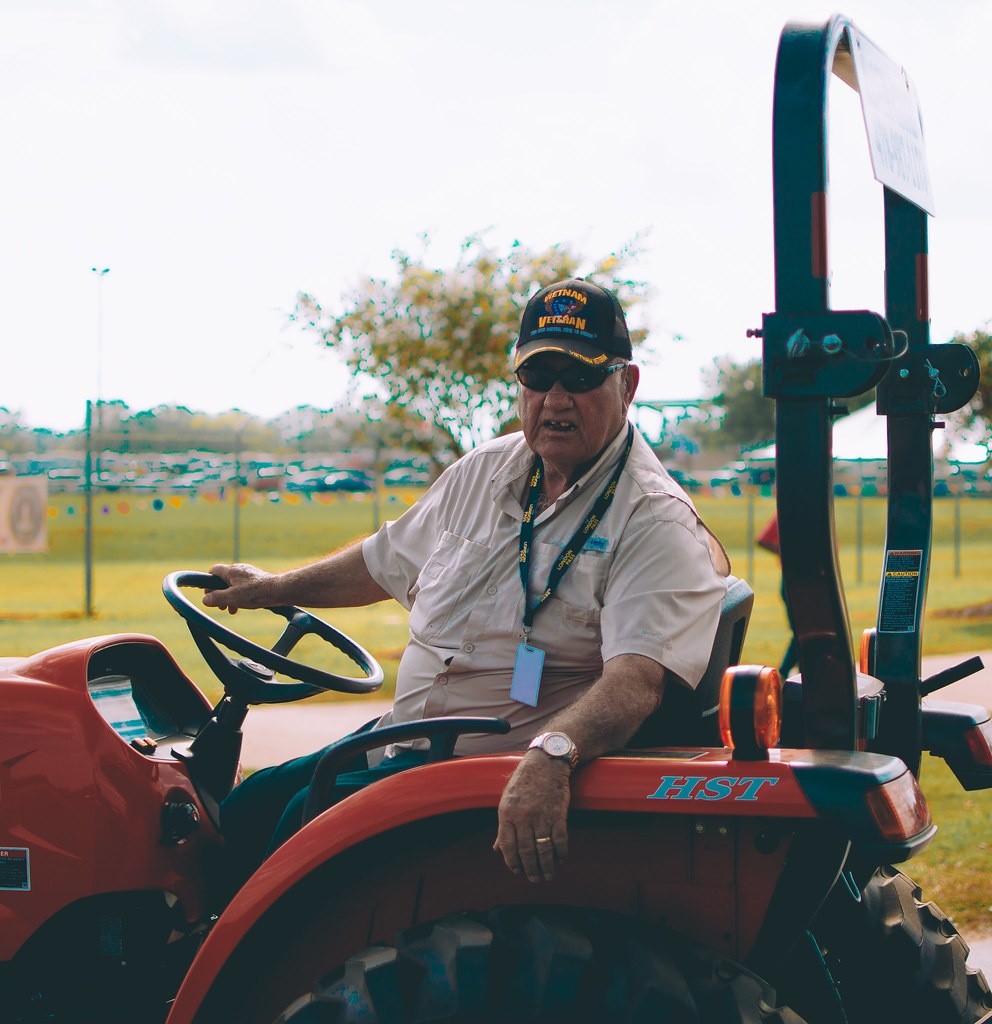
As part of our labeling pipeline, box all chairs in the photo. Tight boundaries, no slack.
[309,570,756,846]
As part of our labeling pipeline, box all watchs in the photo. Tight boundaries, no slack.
[527,732,579,776]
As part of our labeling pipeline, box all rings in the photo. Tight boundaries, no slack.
[536,836,551,844]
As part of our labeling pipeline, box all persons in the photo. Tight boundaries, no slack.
[757,511,799,685]
[201,277,727,1024]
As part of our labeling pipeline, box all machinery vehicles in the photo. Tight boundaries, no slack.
[0,10,992,1024]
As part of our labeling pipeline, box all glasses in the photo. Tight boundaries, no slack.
[516,362,628,393]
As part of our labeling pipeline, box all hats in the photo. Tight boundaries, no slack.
[515,279,632,372]
